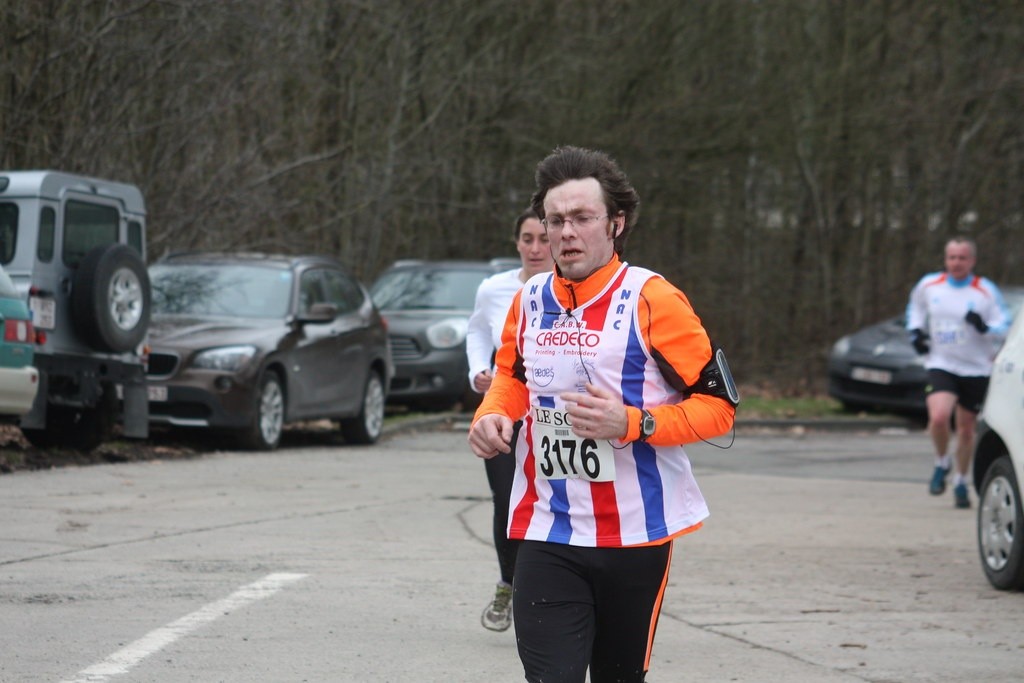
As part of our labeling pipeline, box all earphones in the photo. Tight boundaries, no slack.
[613,224,619,239]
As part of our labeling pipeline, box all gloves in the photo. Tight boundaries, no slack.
[911,329,930,354]
[964,310,988,333]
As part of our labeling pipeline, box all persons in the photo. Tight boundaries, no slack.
[905,235,1012,508]
[468,149,736,683]
[466,209,555,632]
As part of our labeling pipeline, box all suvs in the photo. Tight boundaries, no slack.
[0,167,152,455]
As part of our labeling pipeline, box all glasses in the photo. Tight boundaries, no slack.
[541,213,614,231]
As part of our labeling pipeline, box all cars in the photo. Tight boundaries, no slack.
[970,309,1023,593]
[823,286,1023,432]
[0,267,44,426]
[112,247,396,455]
[368,255,524,413]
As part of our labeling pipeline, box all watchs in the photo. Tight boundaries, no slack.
[633,410,656,444]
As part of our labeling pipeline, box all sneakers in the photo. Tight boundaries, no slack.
[928,459,953,495]
[952,482,970,508]
[480,580,514,632]
